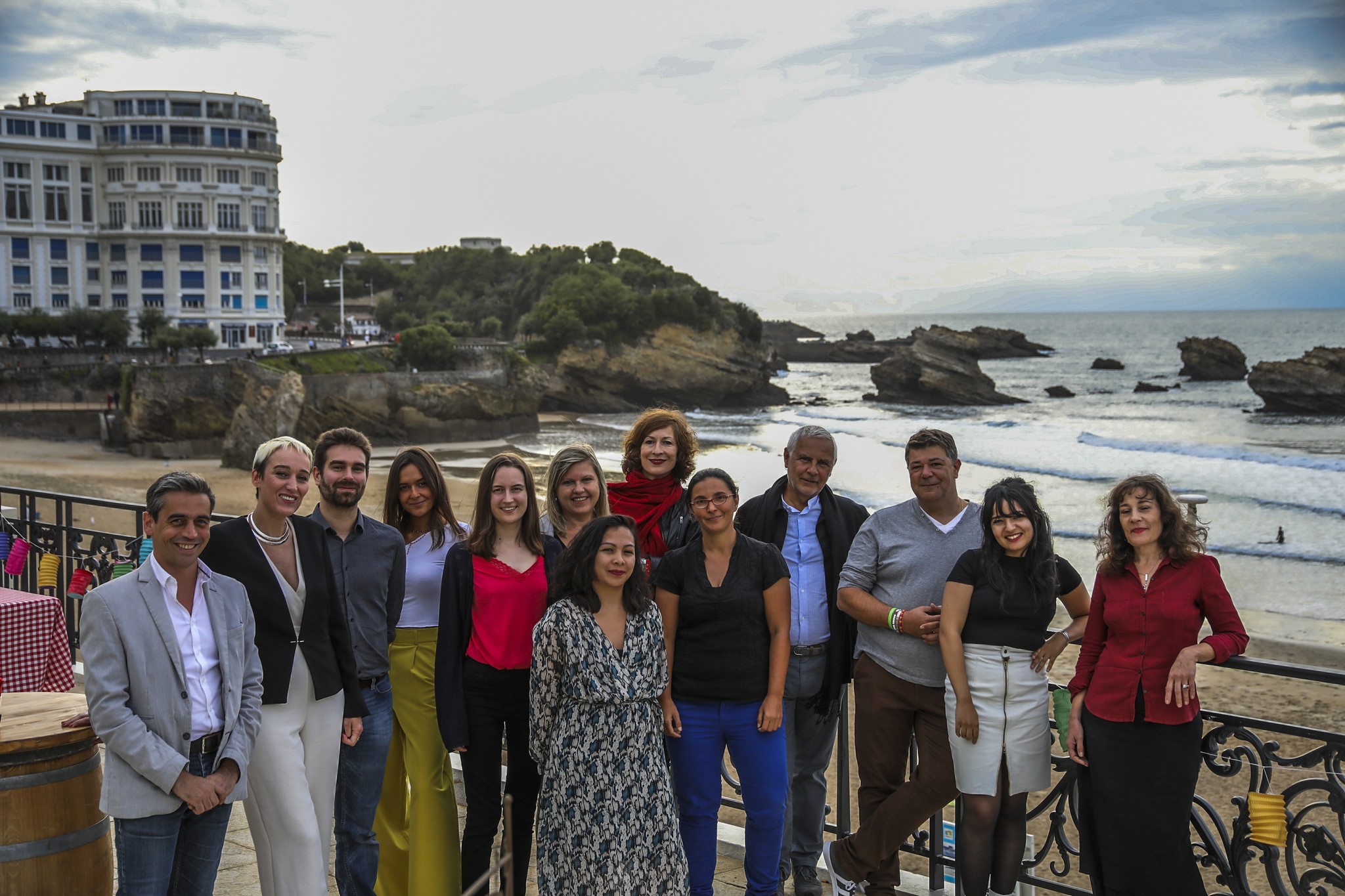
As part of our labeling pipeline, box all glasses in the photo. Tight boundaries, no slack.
[690,493,736,509]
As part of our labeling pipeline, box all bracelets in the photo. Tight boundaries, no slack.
[1056,630,1069,647]
[888,608,906,633]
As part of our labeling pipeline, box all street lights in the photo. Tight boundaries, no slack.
[323,264,344,338]
[298,276,307,304]
[366,278,373,306]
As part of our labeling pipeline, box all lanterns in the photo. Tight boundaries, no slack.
[0,529,157,600]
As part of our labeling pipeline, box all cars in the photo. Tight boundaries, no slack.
[263,341,293,356]
[7,339,149,348]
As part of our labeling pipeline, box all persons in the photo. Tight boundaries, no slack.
[437,454,571,896]
[58,436,369,895]
[732,425,869,896]
[648,467,792,896]
[600,406,704,606]
[937,475,1091,896]
[113,389,121,409]
[528,441,614,613]
[106,392,113,410]
[302,427,408,896]
[0,320,403,370]
[824,430,995,896]
[1065,474,1250,896]
[77,470,264,894]
[375,449,472,892]
[526,513,690,896]
[1275,526,1285,544]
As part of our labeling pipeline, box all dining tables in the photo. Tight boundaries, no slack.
[0,587,61,693]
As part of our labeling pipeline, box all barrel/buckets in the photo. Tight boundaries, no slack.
[0,688,115,896]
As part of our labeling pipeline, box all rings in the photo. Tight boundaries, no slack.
[1182,684,1190,690]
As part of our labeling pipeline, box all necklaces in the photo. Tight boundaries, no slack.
[403,524,435,556]
[1137,551,1166,586]
[246,513,290,547]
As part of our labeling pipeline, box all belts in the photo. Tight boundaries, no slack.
[790,644,827,657]
[359,676,379,689]
[189,726,224,755]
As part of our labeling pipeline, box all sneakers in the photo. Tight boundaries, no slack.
[822,841,866,896]
[791,864,823,896]
[775,879,784,896]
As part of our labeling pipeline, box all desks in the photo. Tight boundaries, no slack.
[0,692,114,896]
